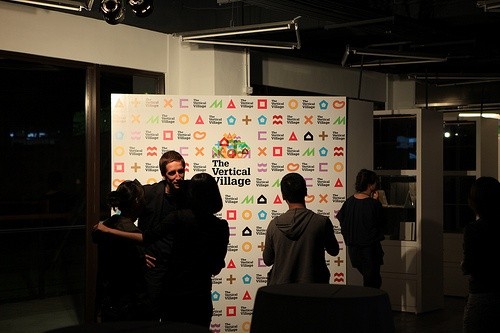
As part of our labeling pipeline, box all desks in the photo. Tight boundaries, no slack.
[249,284,395,333]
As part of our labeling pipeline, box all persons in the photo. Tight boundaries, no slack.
[459,176,500,333]
[263,172,339,286]
[89,151,230,328]
[336,168,394,289]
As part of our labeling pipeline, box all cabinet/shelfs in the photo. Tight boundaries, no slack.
[374,110,443,315]
[444,116,497,295]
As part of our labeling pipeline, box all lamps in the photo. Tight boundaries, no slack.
[101,0,153,25]
